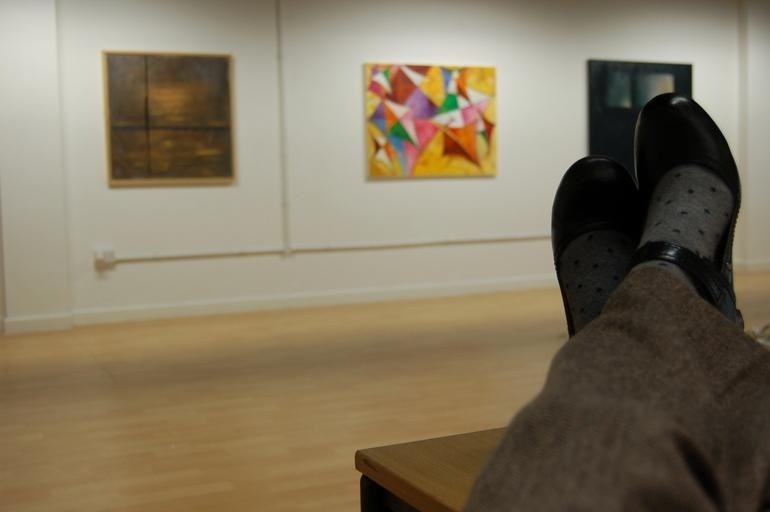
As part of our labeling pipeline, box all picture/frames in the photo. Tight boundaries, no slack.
[101,49,237,188]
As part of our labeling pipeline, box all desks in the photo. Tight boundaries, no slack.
[354,426,512,511]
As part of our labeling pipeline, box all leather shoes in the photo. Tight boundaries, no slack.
[551,155,640,335]
[634,91,744,334]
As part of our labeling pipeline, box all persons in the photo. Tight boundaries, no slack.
[457,92,769,510]
